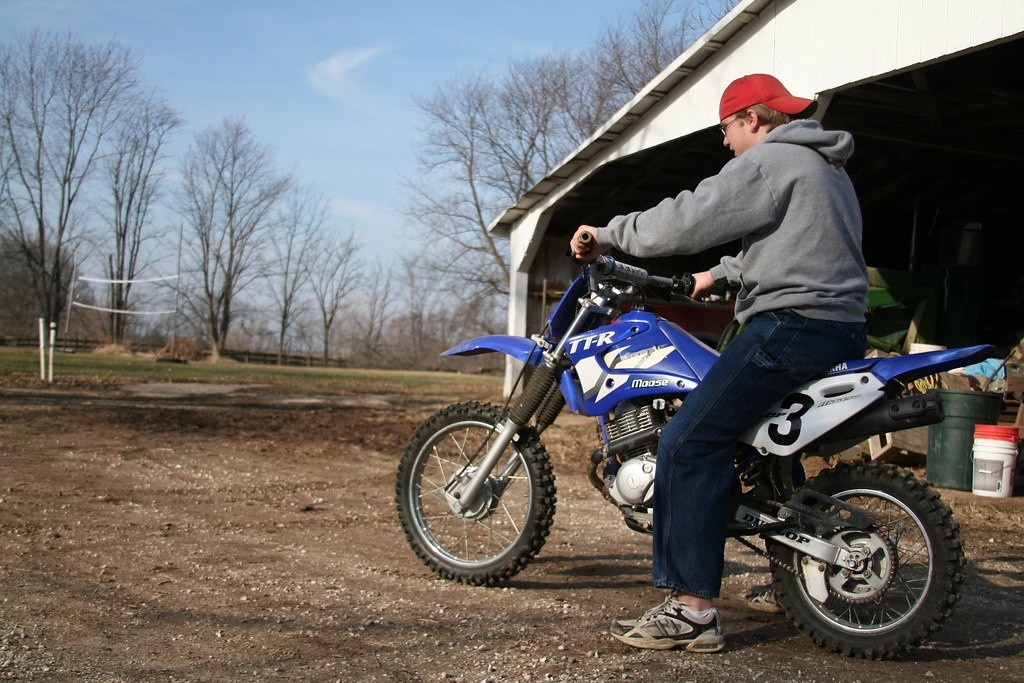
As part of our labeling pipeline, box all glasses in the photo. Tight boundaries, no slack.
[719,115,741,136]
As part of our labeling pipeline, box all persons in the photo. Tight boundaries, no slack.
[571,73,869,656]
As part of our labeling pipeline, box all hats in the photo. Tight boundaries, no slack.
[719,74,818,122]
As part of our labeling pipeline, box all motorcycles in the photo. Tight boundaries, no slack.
[395,230,995,658]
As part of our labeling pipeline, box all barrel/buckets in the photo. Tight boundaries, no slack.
[926,389,1004,492]
[970,424,1020,498]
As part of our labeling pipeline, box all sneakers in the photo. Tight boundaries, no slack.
[745,584,787,614]
[609,594,724,652]
